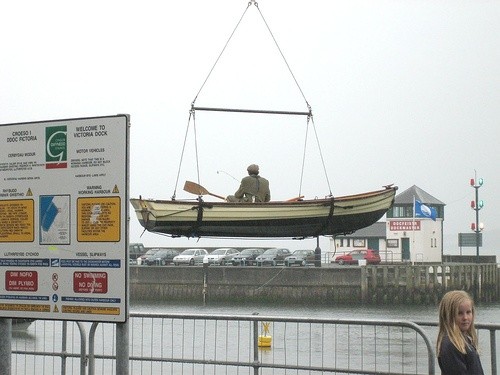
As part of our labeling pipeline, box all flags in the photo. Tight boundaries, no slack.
[415,200,436,221]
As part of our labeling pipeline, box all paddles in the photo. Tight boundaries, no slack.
[183,180,227,201]
[288,196,304,201]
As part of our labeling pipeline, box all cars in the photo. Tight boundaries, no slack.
[255,248,291,267]
[203,248,241,266]
[231,248,265,266]
[172,248,208,266]
[129,242,180,266]
[284,249,315,267]
[335,249,381,266]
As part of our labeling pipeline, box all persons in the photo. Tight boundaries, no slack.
[226,164,270,203]
[436,291,484,375]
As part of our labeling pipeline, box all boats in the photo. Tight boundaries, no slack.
[129,182,400,239]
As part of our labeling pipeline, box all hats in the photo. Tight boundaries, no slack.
[247,164,259,172]
